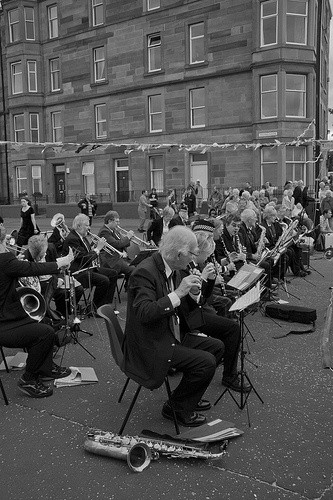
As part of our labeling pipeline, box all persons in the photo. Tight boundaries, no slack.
[0,176,333,398]
[123,225,225,428]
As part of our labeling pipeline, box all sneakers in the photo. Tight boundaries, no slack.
[17,375,53,398]
[40,361,71,378]
[222,374,251,393]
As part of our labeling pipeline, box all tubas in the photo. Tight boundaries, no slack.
[16,259,47,323]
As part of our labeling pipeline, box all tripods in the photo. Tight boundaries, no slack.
[212,209,325,429]
[52,252,99,367]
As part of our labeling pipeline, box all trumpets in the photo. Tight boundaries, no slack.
[87,230,128,259]
[50,213,70,241]
[116,225,150,247]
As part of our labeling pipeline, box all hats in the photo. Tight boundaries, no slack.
[193,219,215,232]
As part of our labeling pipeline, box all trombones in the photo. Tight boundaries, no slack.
[5,234,27,253]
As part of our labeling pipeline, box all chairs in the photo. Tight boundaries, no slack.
[97,305,181,437]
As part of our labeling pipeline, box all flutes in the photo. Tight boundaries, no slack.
[235,232,247,264]
[220,236,237,274]
[211,252,227,297]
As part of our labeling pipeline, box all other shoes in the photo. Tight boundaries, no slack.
[302,269,311,274]
[138,228,146,233]
[294,271,308,277]
[262,295,279,301]
[269,284,277,289]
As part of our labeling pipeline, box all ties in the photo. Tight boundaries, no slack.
[83,237,90,253]
[167,272,181,343]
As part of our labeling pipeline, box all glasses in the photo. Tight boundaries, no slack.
[177,249,199,258]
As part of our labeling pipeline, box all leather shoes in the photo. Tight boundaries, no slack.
[163,402,206,427]
[194,400,211,410]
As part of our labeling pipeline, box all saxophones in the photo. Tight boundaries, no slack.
[83,428,228,473]
[256,216,298,262]
